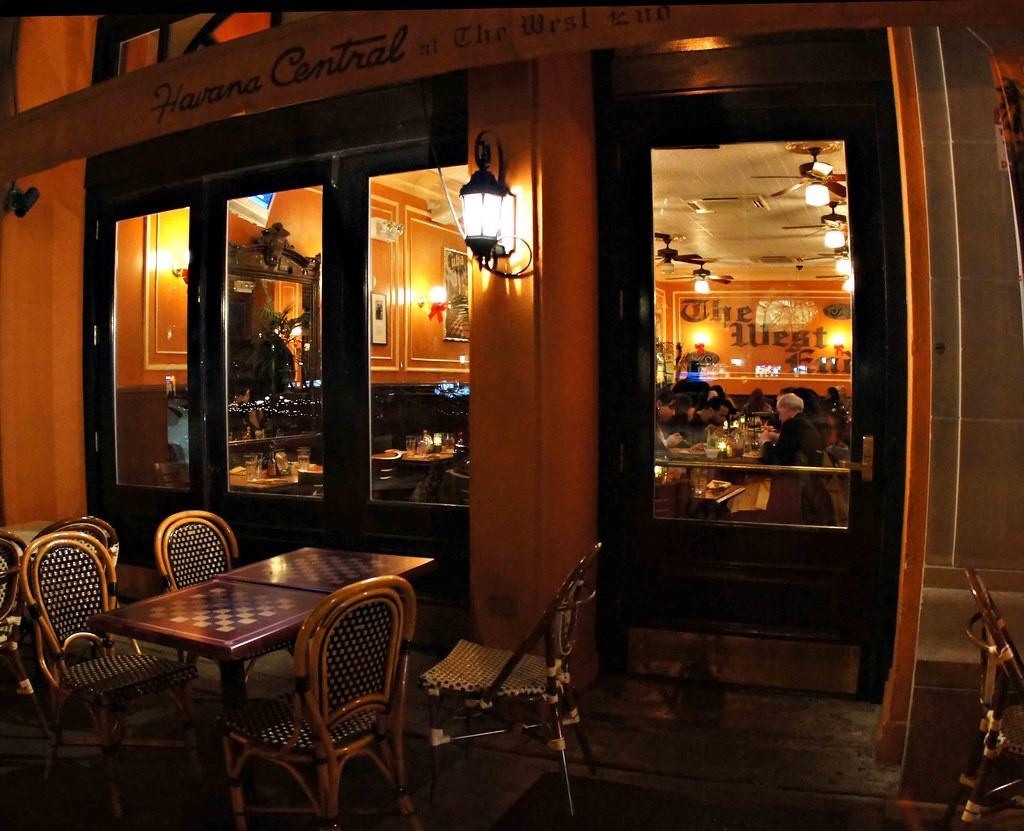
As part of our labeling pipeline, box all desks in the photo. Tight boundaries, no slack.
[87,548,437,712]
[1,521,63,557]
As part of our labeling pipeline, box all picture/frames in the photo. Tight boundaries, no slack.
[370,292,388,345]
[443,247,471,341]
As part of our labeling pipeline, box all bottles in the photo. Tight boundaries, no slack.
[456,432,464,444]
[268,452,276,476]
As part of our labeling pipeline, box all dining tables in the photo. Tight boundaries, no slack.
[655,423,780,519]
[230,454,324,495]
[385,445,458,469]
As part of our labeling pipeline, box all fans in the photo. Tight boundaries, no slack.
[649,235,734,285]
[752,141,852,280]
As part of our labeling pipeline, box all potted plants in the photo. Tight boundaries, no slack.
[231,279,319,395]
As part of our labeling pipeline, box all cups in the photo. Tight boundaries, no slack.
[705,449,718,458]
[244,452,263,482]
[405,431,455,453]
[735,438,744,456]
[296,447,310,471]
[690,468,707,494]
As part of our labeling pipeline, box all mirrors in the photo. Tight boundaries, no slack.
[228,225,326,449]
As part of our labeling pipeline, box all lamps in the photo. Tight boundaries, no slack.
[805,185,853,292]
[461,128,537,279]
[8,181,38,217]
[660,259,709,293]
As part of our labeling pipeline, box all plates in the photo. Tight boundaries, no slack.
[372,452,401,460]
[743,453,762,459]
[680,449,704,455]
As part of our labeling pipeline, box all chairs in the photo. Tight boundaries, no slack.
[799,420,852,526]
[752,411,778,426]
[0,509,602,819]
[943,569,1024,831]
[378,444,468,506]
[297,469,325,496]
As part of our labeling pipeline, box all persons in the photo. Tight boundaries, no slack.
[655,361,852,467]
[228,384,265,433]
